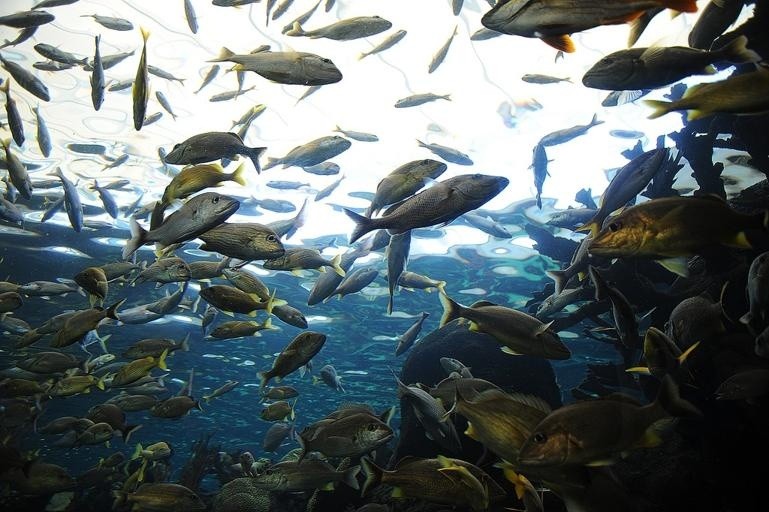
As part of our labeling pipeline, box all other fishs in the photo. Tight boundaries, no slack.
[0,0,768,512]
